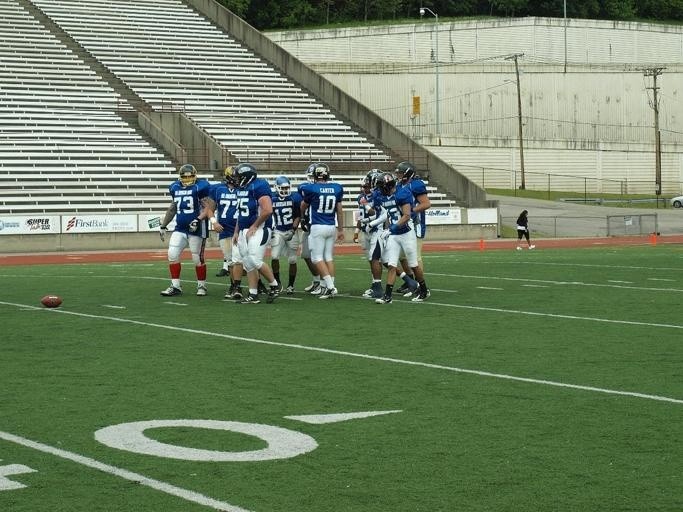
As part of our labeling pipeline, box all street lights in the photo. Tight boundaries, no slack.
[420,6,440,135]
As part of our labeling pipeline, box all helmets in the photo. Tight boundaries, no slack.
[275,176,291,197]
[178,164,197,186]
[363,169,396,196]
[222,164,258,190]
[306,162,330,184]
[395,161,416,185]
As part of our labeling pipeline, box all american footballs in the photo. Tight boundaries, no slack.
[40,295,63,307]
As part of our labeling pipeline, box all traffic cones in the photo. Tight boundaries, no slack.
[478,239,484,250]
[650,234,658,247]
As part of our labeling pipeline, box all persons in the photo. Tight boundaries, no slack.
[159,161,431,304]
[516,210,535,251]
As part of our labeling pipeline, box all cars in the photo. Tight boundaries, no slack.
[669,195,682,208]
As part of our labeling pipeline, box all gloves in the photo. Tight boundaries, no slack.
[189,218,201,233]
[160,226,166,242]
[300,218,308,232]
[381,229,391,240]
[284,229,295,241]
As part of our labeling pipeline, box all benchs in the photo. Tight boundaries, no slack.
[0,1,293,87]
[0,87,460,213]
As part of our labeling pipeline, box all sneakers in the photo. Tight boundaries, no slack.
[305,281,338,299]
[160,282,184,296]
[287,285,296,296]
[225,278,283,304]
[197,283,208,296]
[216,269,230,277]
[363,282,431,304]
